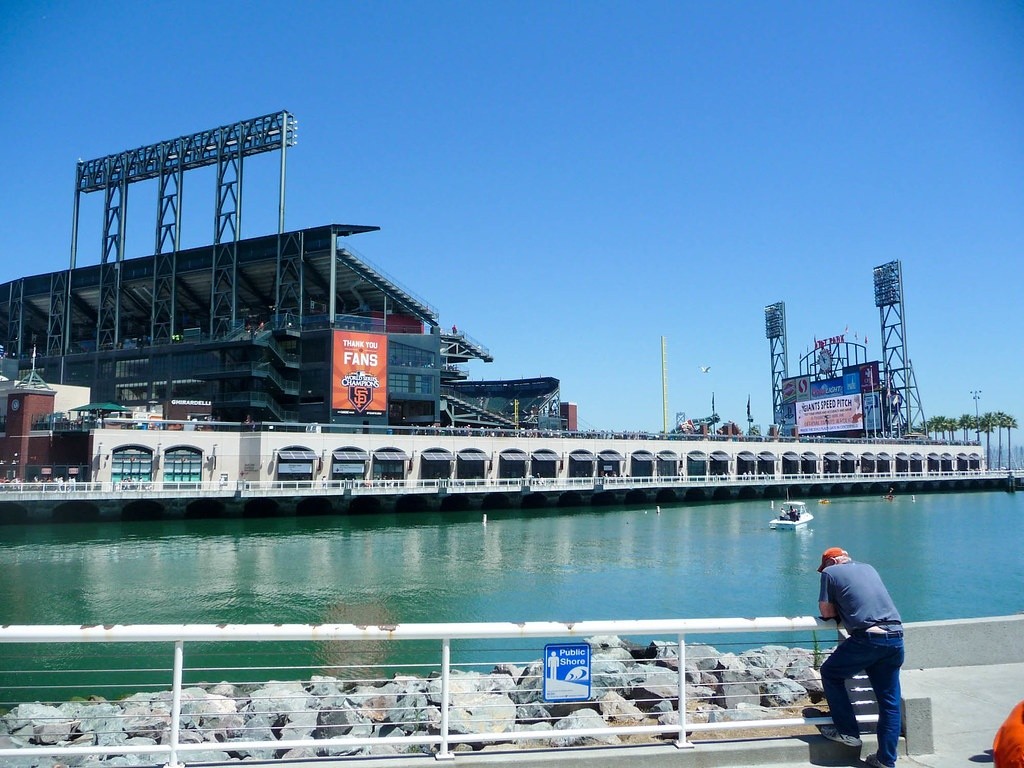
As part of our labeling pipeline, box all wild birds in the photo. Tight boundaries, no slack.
[701,366,711,373]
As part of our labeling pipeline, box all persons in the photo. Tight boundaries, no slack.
[451,324,459,336]
[778,505,798,520]
[245,322,266,333]
[380,466,1023,488]
[0,476,149,490]
[818,547,905,768]
[96,417,103,429]
[409,421,986,448]
[389,354,462,373]
[322,475,328,488]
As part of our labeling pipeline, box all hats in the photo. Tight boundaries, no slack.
[817,547,847,573]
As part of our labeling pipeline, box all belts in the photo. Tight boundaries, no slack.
[852,631,903,638]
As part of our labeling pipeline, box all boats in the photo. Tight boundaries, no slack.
[769,501,815,530]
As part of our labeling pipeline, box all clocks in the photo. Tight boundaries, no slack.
[817,349,833,374]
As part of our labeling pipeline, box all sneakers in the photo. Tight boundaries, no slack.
[820,725,863,747]
[866,753,896,768]
